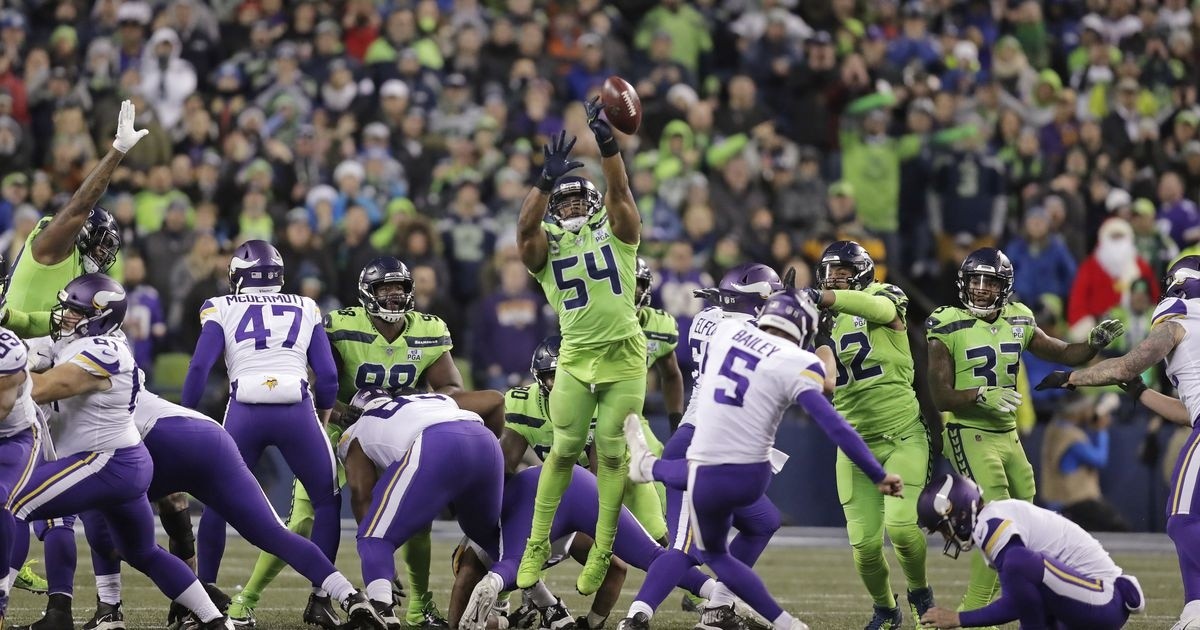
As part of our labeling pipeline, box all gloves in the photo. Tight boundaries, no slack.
[1089,319,1124,350]
[693,288,720,307]
[1034,371,1076,391]
[1117,374,1147,400]
[584,95,620,157]
[976,385,1023,412]
[112,99,149,154]
[534,129,584,193]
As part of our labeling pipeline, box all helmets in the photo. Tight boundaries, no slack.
[916,471,983,550]
[635,256,653,309]
[530,336,561,395]
[719,240,876,350]
[57,273,127,338]
[1162,238,1200,301]
[358,255,417,323]
[958,247,1016,317]
[228,240,285,293]
[75,206,121,269]
[549,175,602,233]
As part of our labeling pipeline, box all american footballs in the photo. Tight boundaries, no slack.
[602,75,644,134]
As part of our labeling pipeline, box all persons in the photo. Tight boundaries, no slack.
[494,331,715,620]
[12,356,387,630]
[226,255,467,630]
[6,275,237,630]
[338,385,511,630]
[1,324,44,621]
[786,241,940,630]
[0,251,68,597]
[172,240,346,630]
[912,473,1146,628]
[448,459,775,630]
[513,97,648,596]
[924,248,1125,629]
[619,291,907,630]
[609,253,720,615]
[615,262,785,629]
[6,97,150,316]
[0,0,1200,392]
[1031,254,1200,630]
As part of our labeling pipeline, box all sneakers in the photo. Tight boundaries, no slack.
[166,538,809,629]
[1167,599,1200,630]
[13,565,50,595]
[82,598,126,630]
[625,413,652,484]
[906,585,941,630]
[864,593,903,630]
